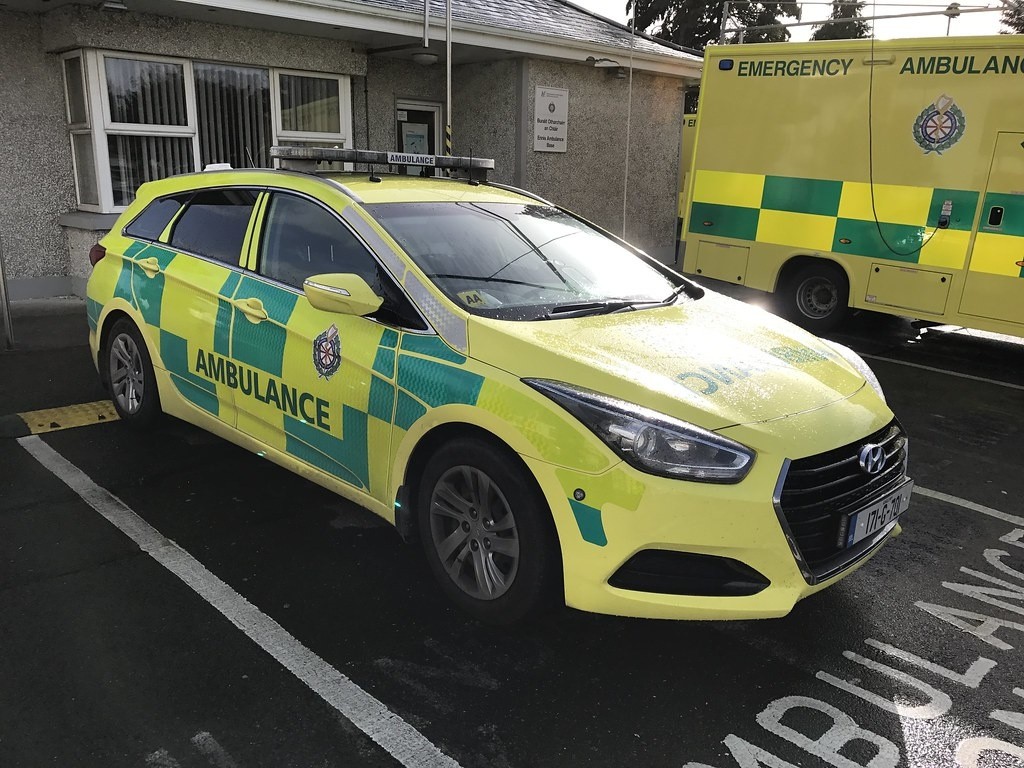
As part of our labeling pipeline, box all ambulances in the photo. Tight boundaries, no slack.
[87,147,915,632]
[676,34,1024,348]
